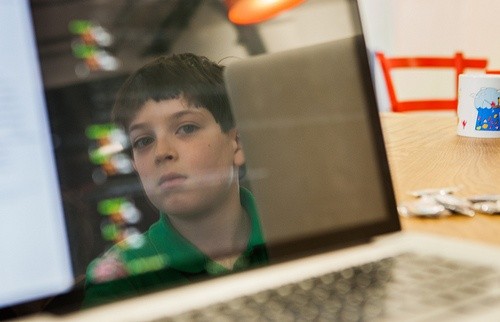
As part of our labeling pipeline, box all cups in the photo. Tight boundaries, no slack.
[455,74,500,139]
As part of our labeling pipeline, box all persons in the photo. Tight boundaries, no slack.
[81,52,273,309]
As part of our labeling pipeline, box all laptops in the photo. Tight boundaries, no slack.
[0,2,500,322]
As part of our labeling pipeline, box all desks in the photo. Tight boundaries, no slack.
[379,110,500,250]
